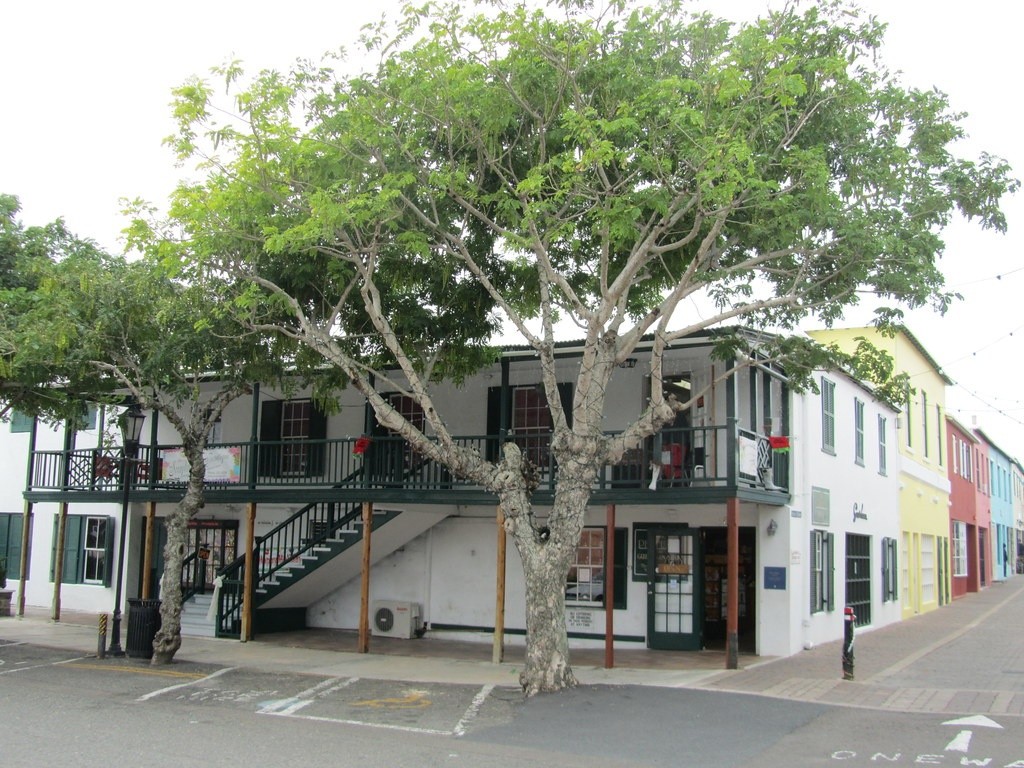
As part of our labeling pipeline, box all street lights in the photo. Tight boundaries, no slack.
[105,403,147,656]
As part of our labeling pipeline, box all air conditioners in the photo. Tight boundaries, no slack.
[371,598,421,641]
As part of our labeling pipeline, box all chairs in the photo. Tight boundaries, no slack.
[662,443,689,488]
[95,455,118,490]
[138,457,164,489]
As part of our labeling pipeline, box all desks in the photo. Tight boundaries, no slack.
[110,456,148,490]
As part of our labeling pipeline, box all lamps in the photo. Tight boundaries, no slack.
[766,518,778,537]
[618,357,638,368]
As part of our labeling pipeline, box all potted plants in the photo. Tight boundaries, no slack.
[0,553,16,618]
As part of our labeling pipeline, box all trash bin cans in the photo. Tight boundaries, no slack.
[126,598,163,659]
